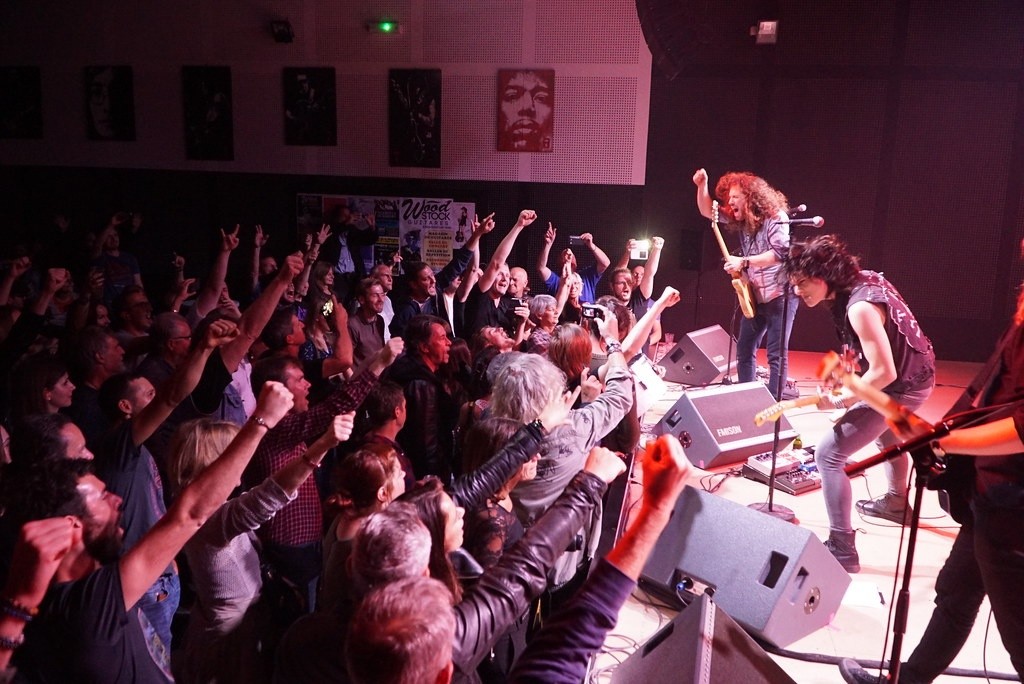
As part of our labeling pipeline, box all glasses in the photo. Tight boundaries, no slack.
[127,301,154,312]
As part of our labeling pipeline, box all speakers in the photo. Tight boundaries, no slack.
[634,0,715,80]
[637,483,852,649]
[652,323,739,386]
[610,592,798,684]
[651,379,799,469]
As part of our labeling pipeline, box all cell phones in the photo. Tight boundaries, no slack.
[570,236,584,245]
[634,241,649,250]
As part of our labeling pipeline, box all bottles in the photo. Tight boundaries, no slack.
[793,436,802,449]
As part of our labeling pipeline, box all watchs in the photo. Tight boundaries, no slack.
[931,441,945,459]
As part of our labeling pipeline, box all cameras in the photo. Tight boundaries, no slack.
[582,305,603,320]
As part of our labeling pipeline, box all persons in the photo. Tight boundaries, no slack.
[1,169,1024,684]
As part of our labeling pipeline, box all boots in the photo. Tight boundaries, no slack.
[854,492,912,525]
[822,529,867,572]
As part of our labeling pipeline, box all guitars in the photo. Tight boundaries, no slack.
[711,200,756,319]
[754,386,847,427]
[815,344,977,524]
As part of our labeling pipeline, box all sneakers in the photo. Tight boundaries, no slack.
[839,657,888,684]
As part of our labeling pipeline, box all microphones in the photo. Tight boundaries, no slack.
[787,205,806,216]
[773,216,824,227]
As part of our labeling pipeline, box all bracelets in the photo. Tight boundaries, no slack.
[606,341,621,351]
[607,349,622,357]
[2,607,32,621]
[9,598,38,616]
[746,256,749,268]
[0,635,24,648]
[251,415,269,431]
[302,455,321,468]
[743,256,745,268]
[170,309,179,313]
[831,398,846,408]
[533,418,549,435]
[592,246,596,252]
